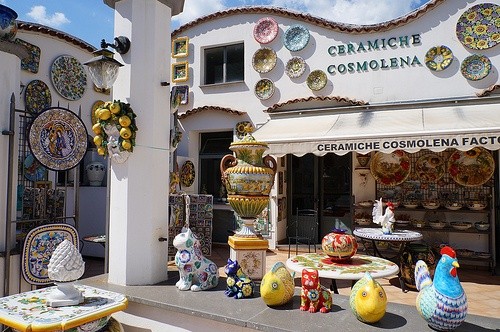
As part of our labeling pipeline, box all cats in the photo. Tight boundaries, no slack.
[223,258,256,299]
[173,226,219,292]
[299,269,332,313]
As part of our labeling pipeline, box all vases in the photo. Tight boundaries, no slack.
[85,161,106,186]
[321,229,357,258]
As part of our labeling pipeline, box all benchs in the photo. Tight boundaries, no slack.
[399,243,436,291]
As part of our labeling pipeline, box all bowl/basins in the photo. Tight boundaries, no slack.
[476,224,489,230]
[357,219,371,225]
[386,203,401,207]
[396,221,409,226]
[360,203,372,207]
[430,223,448,230]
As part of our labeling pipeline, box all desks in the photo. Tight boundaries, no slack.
[285,253,400,295]
[353,227,423,262]
[0,284,129,332]
[82,235,106,248]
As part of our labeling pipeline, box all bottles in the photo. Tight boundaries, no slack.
[86,161,107,186]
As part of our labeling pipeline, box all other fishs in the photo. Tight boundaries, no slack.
[260,262,296,306]
[349,271,387,323]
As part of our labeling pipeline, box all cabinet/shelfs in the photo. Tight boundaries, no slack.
[352,206,491,267]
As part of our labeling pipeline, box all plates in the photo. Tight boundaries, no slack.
[423,205,439,209]
[446,206,462,210]
[452,225,471,230]
[404,204,418,208]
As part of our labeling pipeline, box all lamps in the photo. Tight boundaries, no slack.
[82,36,131,92]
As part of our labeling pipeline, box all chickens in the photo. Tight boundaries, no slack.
[372,197,396,234]
[414,245,468,331]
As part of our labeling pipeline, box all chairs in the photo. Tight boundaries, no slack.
[288,207,320,257]
